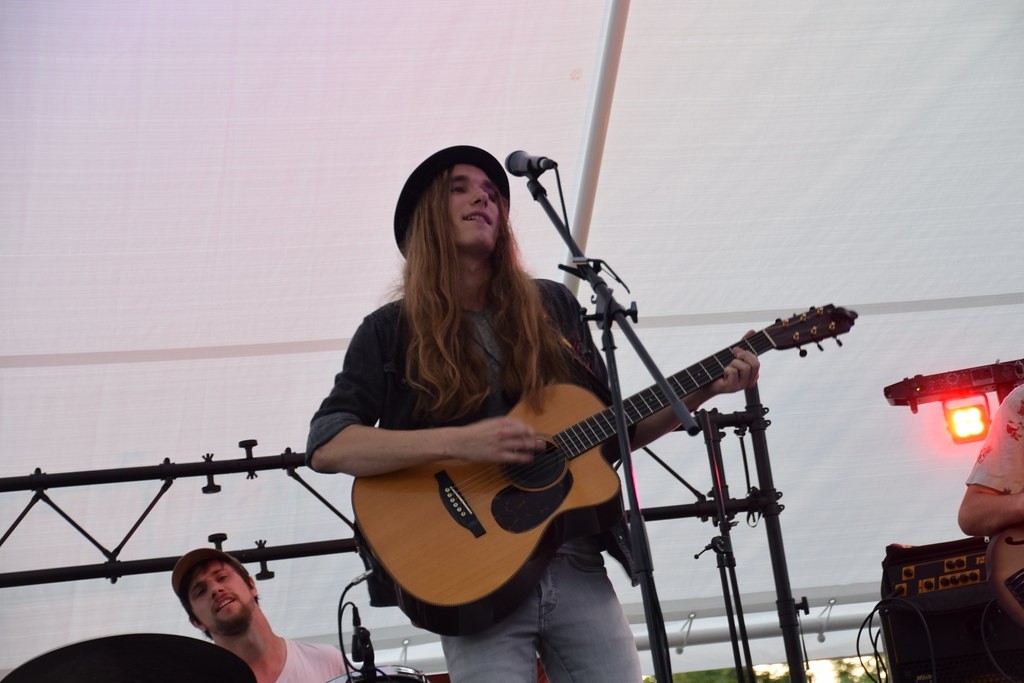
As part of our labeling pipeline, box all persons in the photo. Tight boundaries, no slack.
[957,383,1024,535]
[171,550,350,683]
[307,144,760,680]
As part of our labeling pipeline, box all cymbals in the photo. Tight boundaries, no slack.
[0,633,257,683]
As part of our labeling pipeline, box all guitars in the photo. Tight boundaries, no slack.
[350,303,859,637]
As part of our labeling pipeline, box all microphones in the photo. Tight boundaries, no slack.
[505,151,557,177]
[352,607,368,661]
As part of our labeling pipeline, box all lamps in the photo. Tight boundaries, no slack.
[941,391,992,442]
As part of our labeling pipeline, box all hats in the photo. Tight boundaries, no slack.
[170,548,243,597]
[394,146,512,261]
